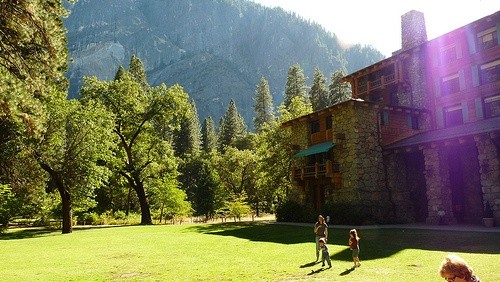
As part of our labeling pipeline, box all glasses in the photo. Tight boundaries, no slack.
[445,275,457,282]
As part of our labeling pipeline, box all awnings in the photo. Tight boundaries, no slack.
[292,141,336,158]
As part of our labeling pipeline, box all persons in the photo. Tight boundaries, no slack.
[317,239,333,268]
[349,229,361,267]
[313,215,328,263]
[438,208,446,223]
[439,255,480,282]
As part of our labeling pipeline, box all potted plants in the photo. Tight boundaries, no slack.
[481,200,496,227]
